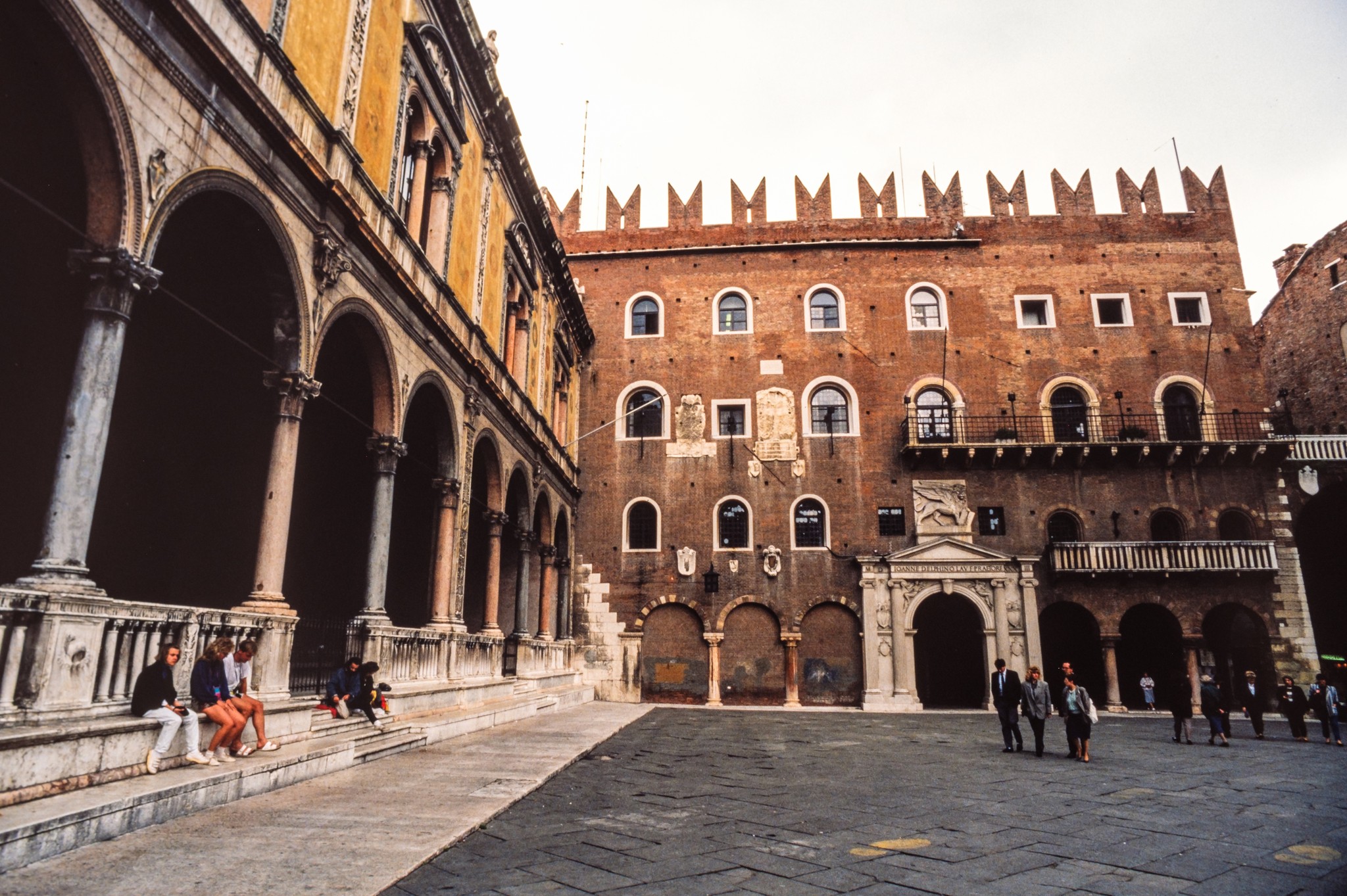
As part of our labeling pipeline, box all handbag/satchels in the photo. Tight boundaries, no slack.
[1087,698,1098,724]
[335,699,350,719]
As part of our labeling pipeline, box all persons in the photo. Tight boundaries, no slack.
[1140,671,1156,710]
[1276,676,1311,742]
[190,637,246,765]
[1200,675,1230,746]
[327,657,385,730]
[222,639,282,756]
[990,658,1024,752]
[1062,674,1091,762]
[1171,678,1192,745]
[1215,680,1232,737]
[1020,665,1054,757]
[1055,661,1084,758]
[1242,671,1266,739]
[1308,673,1344,747]
[130,643,209,774]
[359,661,385,716]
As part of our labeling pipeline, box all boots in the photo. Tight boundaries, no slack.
[1075,738,1082,761]
[1084,739,1090,763]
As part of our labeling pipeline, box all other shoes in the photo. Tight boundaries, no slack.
[1326,738,1331,744]
[1336,741,1344,746]
[374,725,389,731]
[1035,744,1045,757]
[215,753,236,762]
[1148,708,1150,710]
[186,748,209,764]
[1258,735,1264,740]
[1152,708,1155,711]
[208,754,219,766]
[1016,743,1023,753]
[1172,736,1229,747]
[1002,747,1014,754]
[147,750,161,774]
[1303,738,1307,741]
[1297,737,1301,741]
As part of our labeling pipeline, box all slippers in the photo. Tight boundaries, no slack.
[255,741,281,751]
[236,745,256,756]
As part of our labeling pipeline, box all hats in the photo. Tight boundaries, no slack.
[1198,675,1212,682]
[1245,671,1256,678]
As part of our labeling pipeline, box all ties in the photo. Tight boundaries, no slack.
[1001,672,1007,693]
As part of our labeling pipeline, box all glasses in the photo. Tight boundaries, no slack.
[223,652,229,656]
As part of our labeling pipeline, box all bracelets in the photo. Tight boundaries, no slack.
[172,708,175,711]
